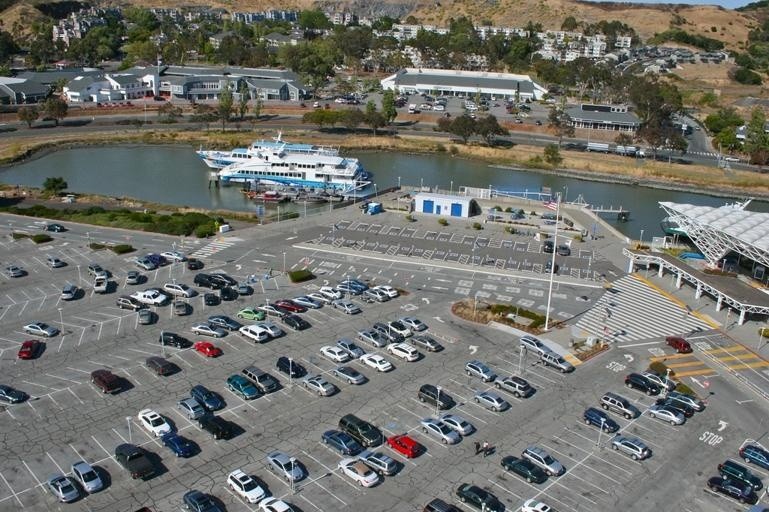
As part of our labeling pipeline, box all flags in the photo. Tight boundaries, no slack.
[543,200,558,211]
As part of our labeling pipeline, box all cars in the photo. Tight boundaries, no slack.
[665,336,692,354]
[584,371,768,504]
[314,96,531,124]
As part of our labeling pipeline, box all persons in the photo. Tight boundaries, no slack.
[481,440,488,458]
[472,441,480,455]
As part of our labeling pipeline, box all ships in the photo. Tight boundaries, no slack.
[196,142,337,170]
[218,155,366,197]
